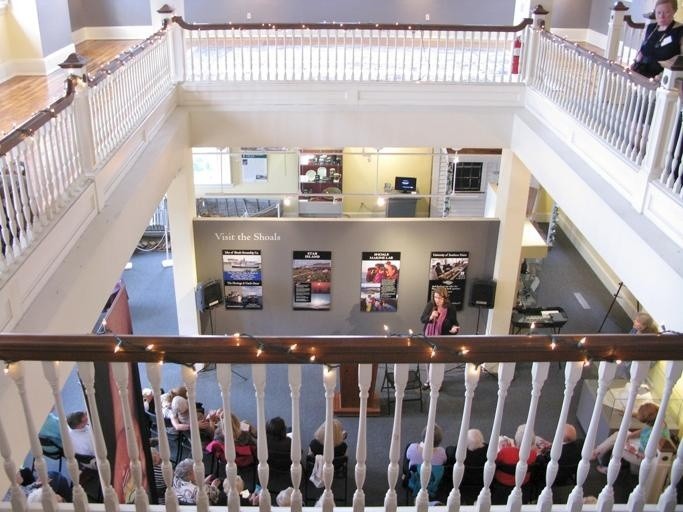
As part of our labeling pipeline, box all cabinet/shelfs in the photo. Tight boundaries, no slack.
[298,150,343,215]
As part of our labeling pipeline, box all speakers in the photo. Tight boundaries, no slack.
[195,279,223,311]
[469,279,497,309]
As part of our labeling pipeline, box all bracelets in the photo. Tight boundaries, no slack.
[635,451,639,456]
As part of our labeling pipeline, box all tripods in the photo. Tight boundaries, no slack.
[198,308,248,381]
[444,305,497,377]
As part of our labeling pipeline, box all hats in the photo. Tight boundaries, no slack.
[172,395,187,414]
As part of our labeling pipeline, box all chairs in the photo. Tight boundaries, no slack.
[379,357,426,416]
[31,387,349,506]
[400,438,586,506]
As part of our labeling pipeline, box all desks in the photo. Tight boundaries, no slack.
[511,306,568,370]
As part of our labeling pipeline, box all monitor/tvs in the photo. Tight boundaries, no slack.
[395,177,416,193]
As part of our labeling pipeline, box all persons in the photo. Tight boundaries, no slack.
[17,406,108,505]
[420,286,460,392]
[435,262,443,276]
[443,259,453,273]
[400,424,585,506]
[613,312,660,380]
[588,404,670,474]
[627,0,683,161]
[365,261,398,312]
[141,387,308,506]
[431,265,438,280]
[310,418,349,477]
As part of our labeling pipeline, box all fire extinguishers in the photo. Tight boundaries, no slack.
[511,35,524,74]
